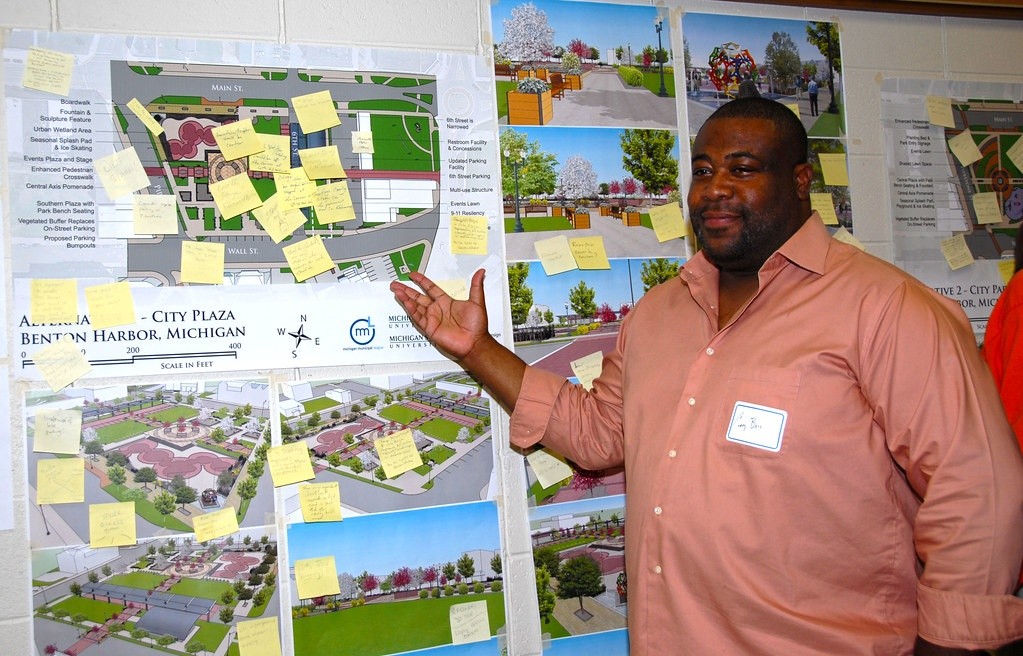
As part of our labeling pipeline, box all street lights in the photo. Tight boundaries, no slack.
[623,175,627,207]
[628,42,631,69]
[564,302,571,336]
[504,148,526,233]
[559,175,563,205]
[433,563,441,590]
[653,14,669,98]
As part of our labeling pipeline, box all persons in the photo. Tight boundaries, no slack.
[391,97,1023,656]
[738,71,761,98]
[808,77,819,117]
[794,75,802,101]
[978,220,1023,448]
